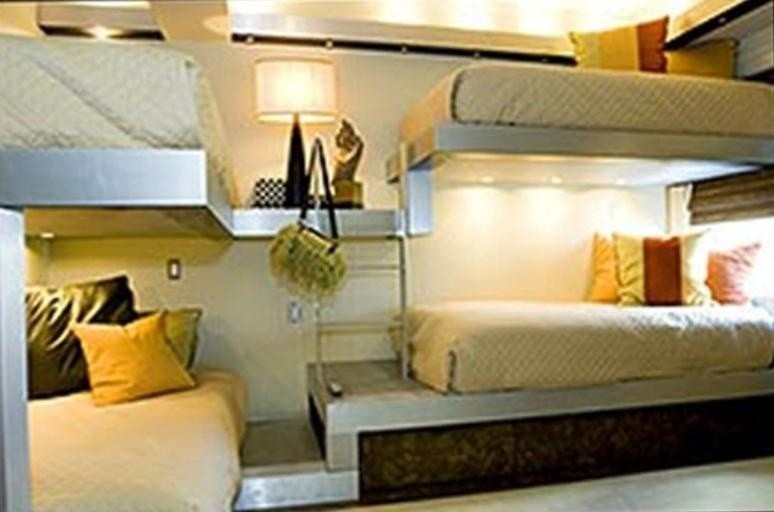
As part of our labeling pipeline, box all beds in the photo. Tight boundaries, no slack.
[0,33,241,512]
[385,60,774,386]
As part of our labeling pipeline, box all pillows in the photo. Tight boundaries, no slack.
[25,273,201,409]
[585,224,758,308]
[569,16,738,79]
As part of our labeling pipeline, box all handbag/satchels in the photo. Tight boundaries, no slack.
[269,138,345,294]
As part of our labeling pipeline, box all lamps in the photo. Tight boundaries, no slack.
[255,57,338,207]
